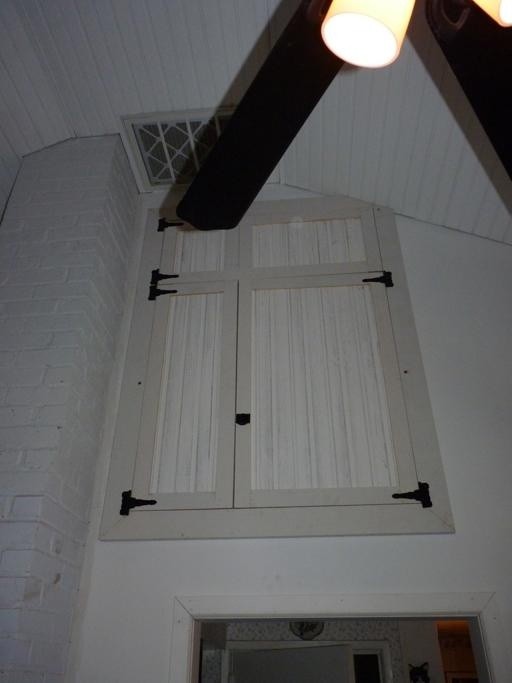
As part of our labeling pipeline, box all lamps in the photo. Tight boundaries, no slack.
[318,0,511,69]
